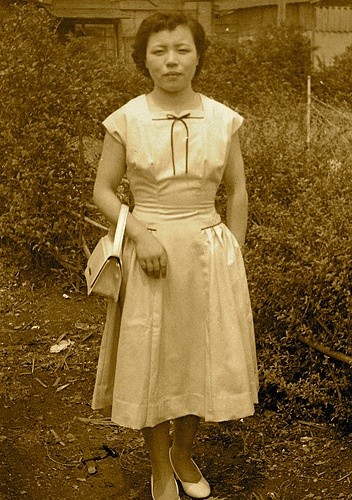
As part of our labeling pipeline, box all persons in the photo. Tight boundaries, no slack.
[92,12,259,499]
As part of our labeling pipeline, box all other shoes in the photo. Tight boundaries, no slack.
[168,447,211,498]
[151,474,181,500]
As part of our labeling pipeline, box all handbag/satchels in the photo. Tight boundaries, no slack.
[85,204,129,303]
